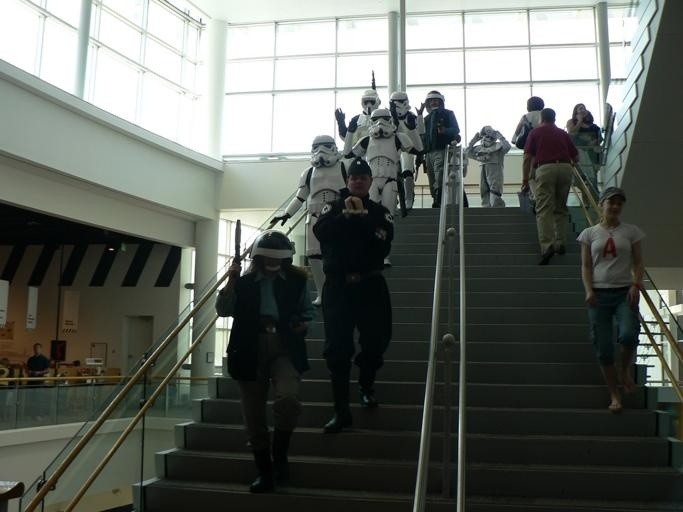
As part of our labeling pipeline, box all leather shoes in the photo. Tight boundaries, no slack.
[538,245,554,265]
[556,245,565,254]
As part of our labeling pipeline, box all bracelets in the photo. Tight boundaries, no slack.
[633,283,642,289]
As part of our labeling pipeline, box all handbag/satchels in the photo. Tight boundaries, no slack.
[517,183,535,216]
[515,116,530,149]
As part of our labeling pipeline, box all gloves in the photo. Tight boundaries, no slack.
[348,115,359,132]
[403,111,416,130]
[335,109,347,136]
[271,214,290,226]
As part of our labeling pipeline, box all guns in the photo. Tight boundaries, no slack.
[230,219,242,287]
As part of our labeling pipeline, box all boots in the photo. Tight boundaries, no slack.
[323,380,353,434]
[311,259,326,306]
[359,374,376,406]
[251,447,272,492]
[271,429,291,471]
[384,257,391,265]
[435,188,441,207]
[404,177,414,210]
[432,197,436,208]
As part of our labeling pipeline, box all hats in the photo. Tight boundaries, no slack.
[348,158,371,174]
[599,186,626,205]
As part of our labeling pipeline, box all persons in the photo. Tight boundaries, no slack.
[583,110,603,202]
[567,103,596,207]
[343,109,425,267]
[449,135,469,208]
[334,90,400,162]
[520,108,580,264]
[511,96,547,205]
[270,134,350,306]
[465,125,511,207]
[415,90,470,208]
[25,343,51,388]
[576,186,645,411]
[312,160,394,433]
[216,230,315,493]
[362,92,425,212]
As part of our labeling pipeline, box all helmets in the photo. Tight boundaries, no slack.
[361,90,381,114]
[249,229,293,270]
[388,92,410,118]
[479,126,496,147]
[368,109,397,139]
[425,90,445,113]
[310,135,337,168]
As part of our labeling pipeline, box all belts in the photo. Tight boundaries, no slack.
[261,327,278,336]
[535,159,570,166]
[323,270,379,284]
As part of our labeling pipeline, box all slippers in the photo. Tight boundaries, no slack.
[617,374,636,394]
[609,402,623,412]
